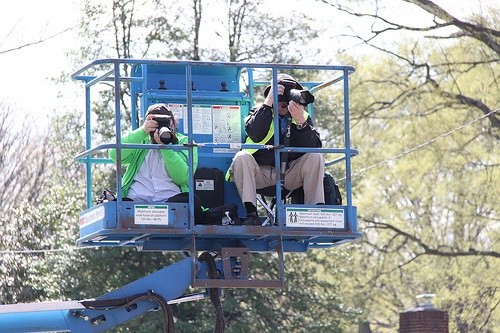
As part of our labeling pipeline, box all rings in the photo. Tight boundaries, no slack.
[292,109,294,111]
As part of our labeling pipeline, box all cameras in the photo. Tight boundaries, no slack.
[278,83,314,106]
[149,115,174,144]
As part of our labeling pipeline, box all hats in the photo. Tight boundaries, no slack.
[263,73,303,100]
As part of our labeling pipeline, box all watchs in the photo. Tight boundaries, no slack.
[296,121,306,129]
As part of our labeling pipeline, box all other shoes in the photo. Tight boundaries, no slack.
[243,212,262,225]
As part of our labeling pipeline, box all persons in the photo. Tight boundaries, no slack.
[225,73,325,225]
[108,103,201,225]
